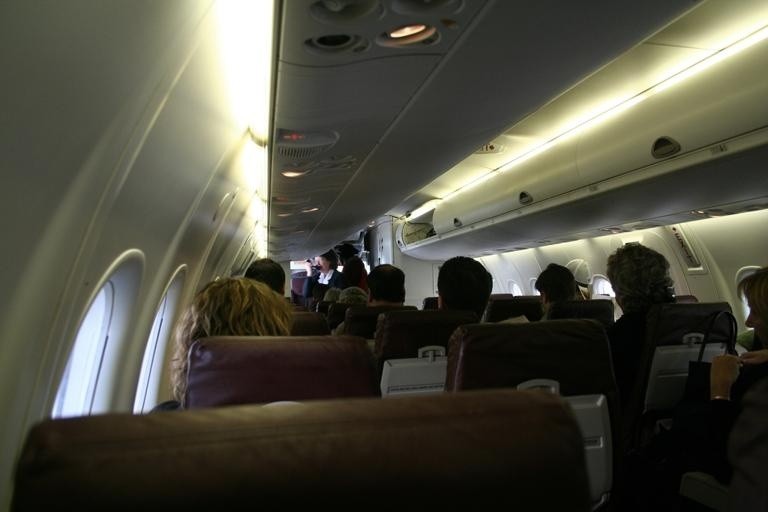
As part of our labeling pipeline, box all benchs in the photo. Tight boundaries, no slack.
[9,295,733,512]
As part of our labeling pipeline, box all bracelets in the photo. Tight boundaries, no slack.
[708,394,732,402]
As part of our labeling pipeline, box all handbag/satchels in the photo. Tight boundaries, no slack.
[685,311,737,392]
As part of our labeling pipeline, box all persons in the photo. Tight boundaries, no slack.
[640,266,768,510]
[602,239,681,410]
[139,274,295,416]
[565,256,594,302]
[225,240,495,352]
[533,263,579,322]
